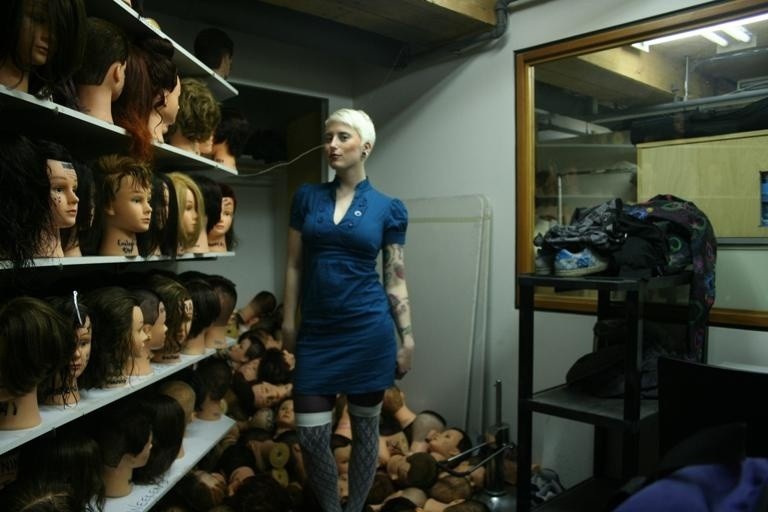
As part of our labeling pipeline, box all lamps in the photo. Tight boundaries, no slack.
[576,57,767,126]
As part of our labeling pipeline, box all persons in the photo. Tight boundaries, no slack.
[0,0,491,512]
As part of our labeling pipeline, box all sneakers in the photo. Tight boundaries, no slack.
[533,250,552,278]
[551,246,608,280]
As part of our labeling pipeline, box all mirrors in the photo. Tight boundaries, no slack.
[510,2,767,336]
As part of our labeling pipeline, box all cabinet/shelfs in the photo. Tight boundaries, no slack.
[1,0,252,511]
[534,172,622,259]
[508,265,712,511]
